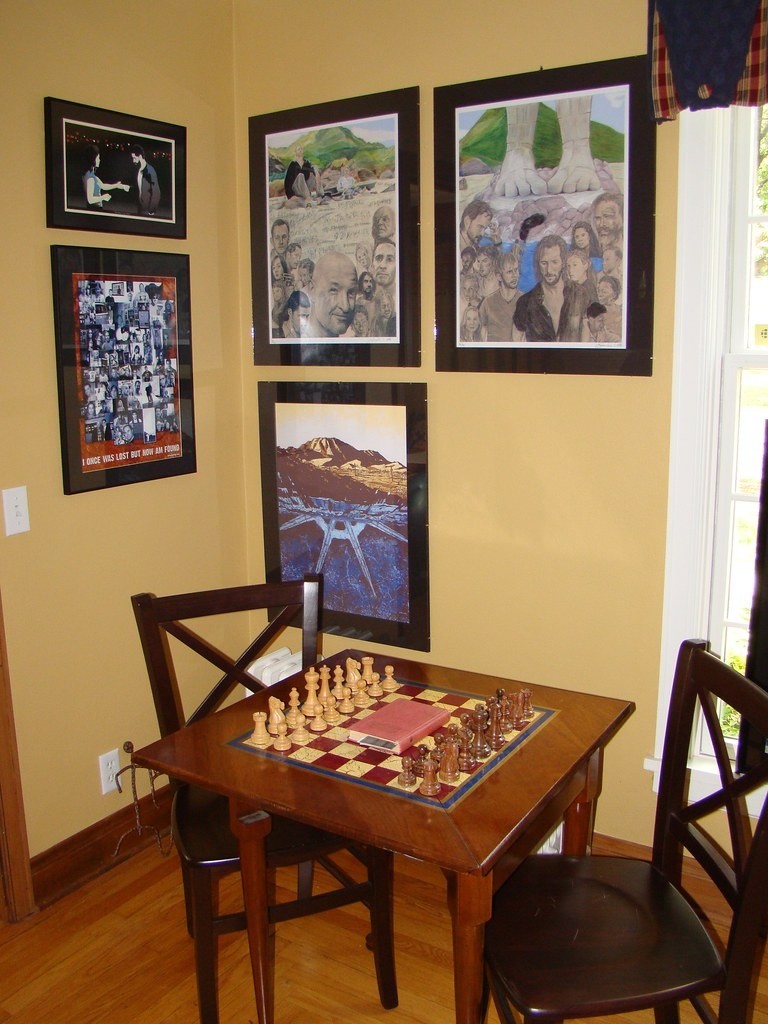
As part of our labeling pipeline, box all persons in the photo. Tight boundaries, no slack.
[75,277,182,448]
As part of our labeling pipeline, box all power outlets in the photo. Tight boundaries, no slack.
[98,748,122,796]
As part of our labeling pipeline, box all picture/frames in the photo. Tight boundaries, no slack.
[44,96,187,241]
[247,86,421,367]
[49,244,197,496]
[257,381,431,653]
[433,54,658,377]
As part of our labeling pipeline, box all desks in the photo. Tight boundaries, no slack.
[131,648,636,1024]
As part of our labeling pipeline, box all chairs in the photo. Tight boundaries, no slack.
[129,575,400,1024]
[485,638,768,1024]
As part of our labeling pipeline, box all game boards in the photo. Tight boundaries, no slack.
[225,672,562,812]
